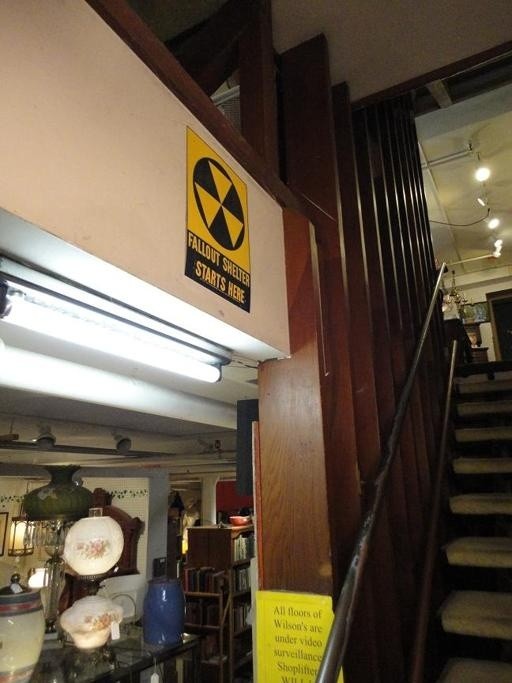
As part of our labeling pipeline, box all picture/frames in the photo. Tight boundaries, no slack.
[0,511,9,557]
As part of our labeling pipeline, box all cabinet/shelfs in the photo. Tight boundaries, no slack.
[30,620,195,683]
[179,524,255,682]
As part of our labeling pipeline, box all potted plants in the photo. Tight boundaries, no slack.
[456,296,485,347]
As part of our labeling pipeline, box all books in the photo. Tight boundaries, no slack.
[179,526,254,668]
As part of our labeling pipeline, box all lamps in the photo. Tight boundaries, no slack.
[0,278,233,383]
[8,516,36,557]
[115,434,130,454]
[36,431,56,450]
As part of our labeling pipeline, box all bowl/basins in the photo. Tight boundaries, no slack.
[229,516,249,526]
